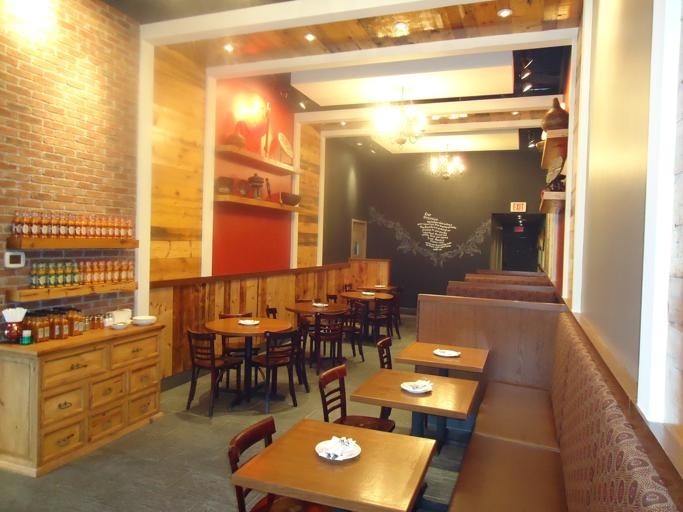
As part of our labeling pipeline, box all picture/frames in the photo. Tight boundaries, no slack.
[510,201,526,213]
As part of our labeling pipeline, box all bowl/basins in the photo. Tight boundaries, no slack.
[281,192,301,206]
[133,315,157,326]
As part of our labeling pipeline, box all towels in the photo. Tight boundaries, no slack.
[326,434,360,457]
[412,379,434,390]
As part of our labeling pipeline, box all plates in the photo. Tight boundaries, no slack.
[278,132,293,160]
[315,439,361,461]
[111,325,127,330]
[433,350,459,357]
[400,381,432,394]
[238,284,387,328]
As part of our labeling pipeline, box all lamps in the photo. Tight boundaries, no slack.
[298,99,311,110]
[519,66,532,80]
[521,81,533,93]
[520,53,534,69]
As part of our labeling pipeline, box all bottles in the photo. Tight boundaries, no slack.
[30,261,81,289]
[78,258,135,285]
[83,313,113,331]
[12,208,134,240]
[22,306,84,342]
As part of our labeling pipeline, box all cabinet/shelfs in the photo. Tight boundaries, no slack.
[1,323,166,479]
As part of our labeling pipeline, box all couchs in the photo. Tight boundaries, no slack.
[447,271,565,303]
[415,293,680,512]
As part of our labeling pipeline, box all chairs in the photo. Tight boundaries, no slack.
[319,364,395,434]
[229,416,331,512]
[376,337,427,429]
[184,281,404,418]
